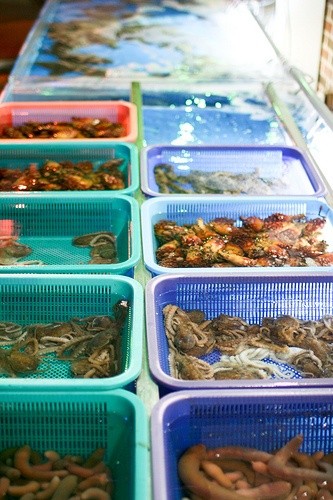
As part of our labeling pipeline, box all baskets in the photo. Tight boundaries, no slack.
[0,100,137,142]
[150,386,333,500]
[0,142,140,194]
[0,194,141,275]
[140,195,333,273]
[0,273,142,389]
[144,274,333,390]
[139,144,327,198]
[0,388,150,500]
